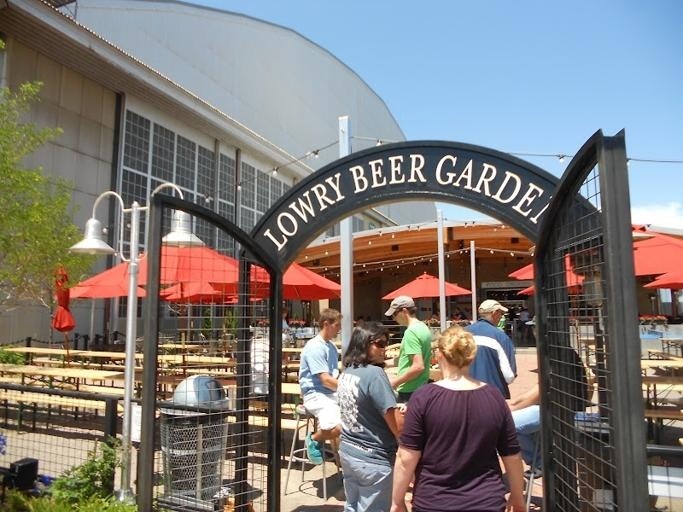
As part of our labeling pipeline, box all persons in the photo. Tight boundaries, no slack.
[337,319,405,512]
[385,295,431,497]
[297,307,343,468]
[497,299,537,347]
[432,298,480,323]
[507,312,589,483]
[462,298,518,402]
[386,323,531,512]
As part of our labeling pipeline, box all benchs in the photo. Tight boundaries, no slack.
[580,336,683,443]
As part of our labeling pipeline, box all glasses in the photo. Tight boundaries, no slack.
[373,339,389,348]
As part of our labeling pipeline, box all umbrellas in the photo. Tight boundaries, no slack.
[380,271,473,319]
[508,221,683,298]
[48,243,343,375]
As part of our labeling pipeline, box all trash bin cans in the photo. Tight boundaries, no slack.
[160,374,232,493]
[575,411,617,502]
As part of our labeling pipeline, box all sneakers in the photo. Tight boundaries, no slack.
[305,431,323,465]
[524,464,542,480]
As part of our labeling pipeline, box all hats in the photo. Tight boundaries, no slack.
[385,296,415,317]
[478,299,509,313]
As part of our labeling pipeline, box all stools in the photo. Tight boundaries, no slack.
[506,428,542,512]
[283,404,343,501]
